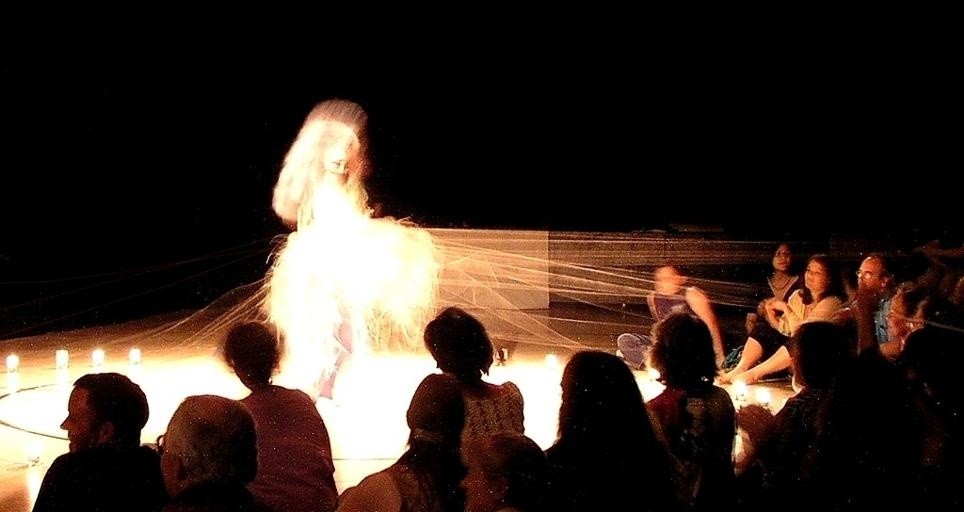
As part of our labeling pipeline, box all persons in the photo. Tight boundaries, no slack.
[720,254,847,387]
[223,322,339,510]
[425,312,522,472]
[745,244,803,327]
[645,314,737,511]
[34,373,170,512]
[741,321,852,512]
[618,265,727,371]
[541,351,693,512]
[880,285,924,359]
[460,434,548,512]
[339,373,464,512]
[849,252,900,352]
[161,396,258,511]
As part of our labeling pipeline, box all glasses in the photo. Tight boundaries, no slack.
[155,436,186,464]
[853,270,879,280]
